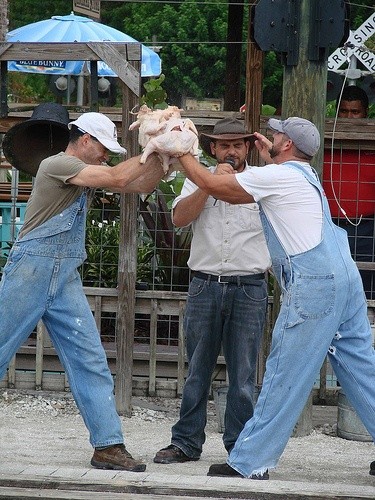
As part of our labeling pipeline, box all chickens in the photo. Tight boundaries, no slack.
[130,105,200,172]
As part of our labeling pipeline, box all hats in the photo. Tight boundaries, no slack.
[68,112,127,154]
[199,118,258,159]
[268,117,320,157]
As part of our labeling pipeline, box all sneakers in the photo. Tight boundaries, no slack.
[90,444,146,471]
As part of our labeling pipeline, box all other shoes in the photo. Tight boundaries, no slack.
[154,444,199,464]
[207,463,245,477]
[369,460,375,476]
[251,469,269,480]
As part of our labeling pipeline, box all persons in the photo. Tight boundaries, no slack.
[324,86,375,299]
[154,118,274,463]
[0,111,172,472]
[172,116,375,480]
[263,84,283,116]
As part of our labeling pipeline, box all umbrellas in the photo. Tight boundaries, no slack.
[7,11,162,104]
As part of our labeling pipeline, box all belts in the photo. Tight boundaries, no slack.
[191,270,265,286]
